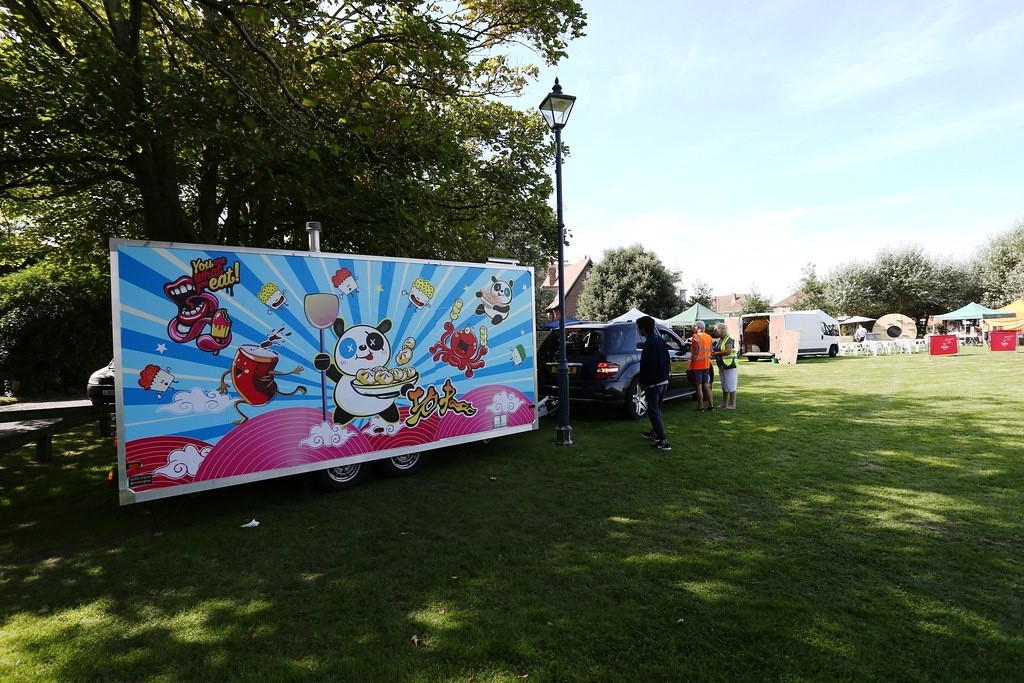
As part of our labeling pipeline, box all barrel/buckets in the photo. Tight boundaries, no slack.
[772,358,778,363]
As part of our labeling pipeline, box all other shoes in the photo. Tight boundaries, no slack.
[726,404,736,410]
[707,407,714,412]
[717,404,728,408]
[693,408,704,413]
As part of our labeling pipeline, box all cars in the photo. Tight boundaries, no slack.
[86,357,115,405]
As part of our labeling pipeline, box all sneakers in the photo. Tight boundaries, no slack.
[642,431,660,442]
[654,441,672,450]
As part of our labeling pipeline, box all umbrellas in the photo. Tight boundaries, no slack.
[839,316,877,325]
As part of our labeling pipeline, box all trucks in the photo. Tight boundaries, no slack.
[724,309,841,362]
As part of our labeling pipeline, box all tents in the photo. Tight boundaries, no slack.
[666,302,725,325]
[985,298,1024,334]
[609,307,670,329]
[541,317,583,328]
[933,302,1018,321]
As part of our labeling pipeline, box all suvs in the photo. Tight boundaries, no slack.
[536,319,719,421]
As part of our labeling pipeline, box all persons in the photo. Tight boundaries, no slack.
[713,323,738,409]
[975,321,990,347]
[688,320,716,412]
[636,317,673,451]
[857,325,868,342]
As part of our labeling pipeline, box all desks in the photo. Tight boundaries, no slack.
[965,334,979,347]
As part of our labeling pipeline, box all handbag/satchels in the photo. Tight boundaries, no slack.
[686,370,698,385]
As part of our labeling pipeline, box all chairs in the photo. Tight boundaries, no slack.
[838,338,925,357]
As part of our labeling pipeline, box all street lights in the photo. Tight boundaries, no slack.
[538,77,576,448]
